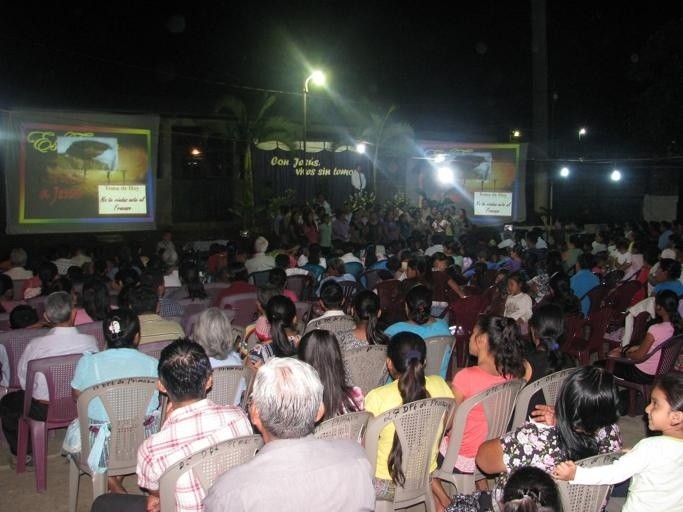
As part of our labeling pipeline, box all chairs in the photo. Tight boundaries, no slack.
[369,259,391,273]
[475,285,502,320]
[285,274,309,300]
[510,366,583,433]
[430,377,528,512]
[184,310,202,338]
[312,411,376,450]
[157,432,266,512]
[137,338,179,361]
[298,263,327,281]
[439,293,490,369]
[376,278,403,312]
[423,334,458,377]
[67,375,169,512]
[206,364,257,411]
[577,281,616,316]
[605,331,683,418]
[485,269,501,284]
[179,294,212,327]
[10,277,29,299]
[362,267,396,292]
[563,312,583,354]
[364,397,457,512]
[336,280,363,312]
[0,327,51,441]
[219,290,262,328]
[549,451,626,512]
[203,280,230,305]
[303,314,358,334]
[292,299,313,336]
[76,318,112,350]
[569,308,626,365]
[245,269,274,289]
[342,261,363,277]
[606,278,642,312]
[16,352,85,489]
[342,344,390,397]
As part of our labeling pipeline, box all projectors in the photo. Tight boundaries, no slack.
[180,243,219,273]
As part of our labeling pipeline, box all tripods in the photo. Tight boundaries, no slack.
[188,272,207,301]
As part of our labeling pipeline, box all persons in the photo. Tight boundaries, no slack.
[592,289,683,385]
[431,313,526,511]
[383,286,451,385]
[243,285,282,342]
[361,331,454,502]
[570,226,608,253]
[297,330,364,429]
[351,163,366,194]
[356,255,418,285]
[536,271,581,316]
[568,253,600,317]
[448,227,569,276]
[165,306,247,415]
[120,269,139,288]
[503,273,533,336]
[269,196,471,254]
[202,356,375,512]
[0,291,99,472]
[628,219,683,256]
[552,373,683,512]
[419,252,469,323]
[127,285,186,345]
[269,267,298,303]
[0,272,28,315]
[62,307,160,496]
[139,270,185,317]
[316,258,356,296]
[503,467,564,512]
[74,279,118,327]
[335,290,391,386]
[3,248,33,279]
[442,366,623,511]
[30,231,119,291]
[245,294,303,398]
[274,253,313,277]
[166,230,244,305]
[600,221,628,289]
[505,303,578,433]
[307,280,346,327]
[90,337,253,512]
[650,256,683,299]
[120,238,166,270]
[627,252,659,308]
[244,236,275,285]
[9,304,38,330]
